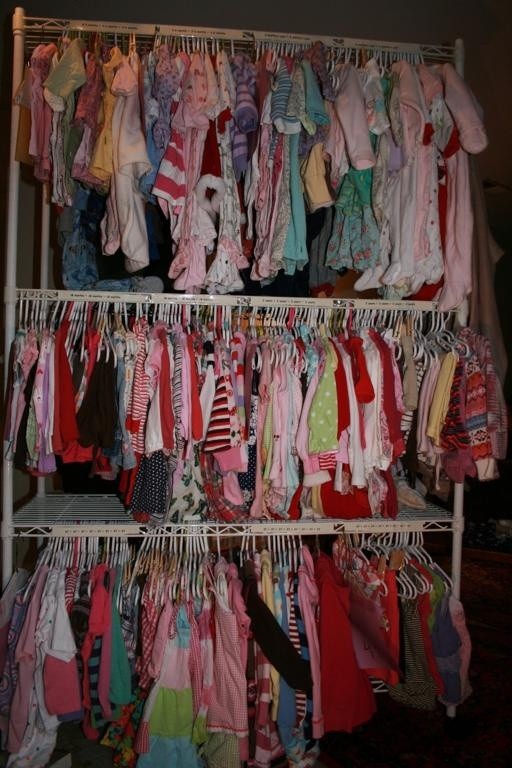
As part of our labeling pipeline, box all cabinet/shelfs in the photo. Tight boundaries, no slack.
[0,6,481,722]
